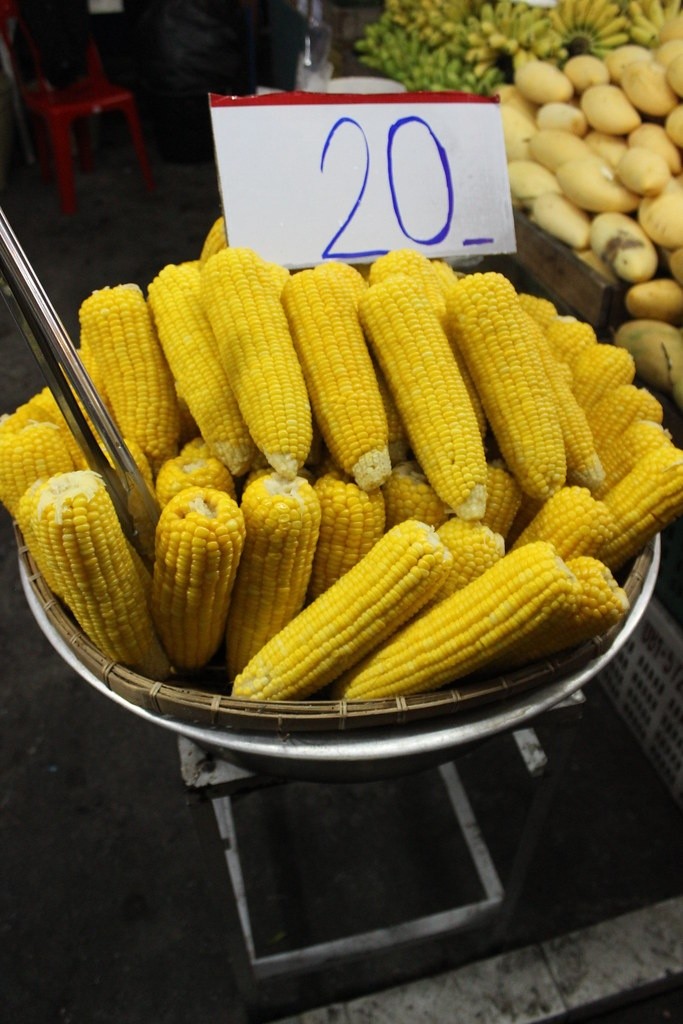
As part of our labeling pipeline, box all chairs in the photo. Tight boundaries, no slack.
[1,0,155,215]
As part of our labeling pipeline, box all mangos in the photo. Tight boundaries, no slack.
[491,19,683,405]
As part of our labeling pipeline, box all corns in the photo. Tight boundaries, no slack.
[1,220,681,699]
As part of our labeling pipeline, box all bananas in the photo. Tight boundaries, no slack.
[354,0,682,96]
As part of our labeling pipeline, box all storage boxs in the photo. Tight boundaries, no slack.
[14,521,662,786]
